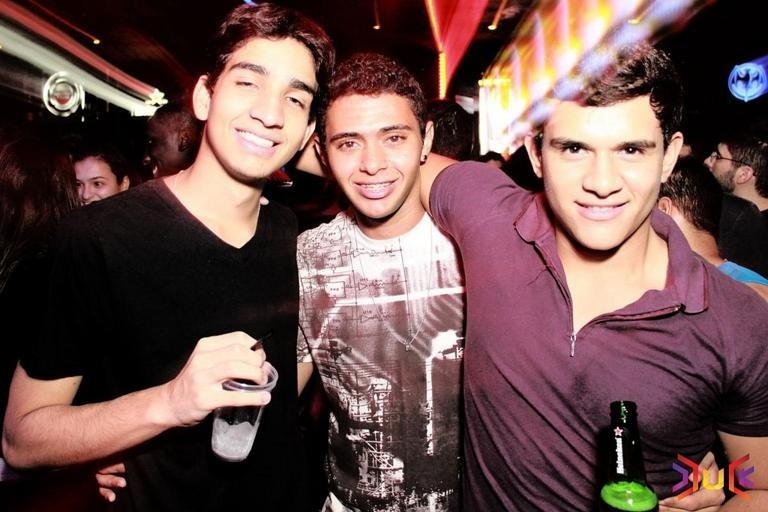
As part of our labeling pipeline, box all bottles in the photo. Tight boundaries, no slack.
[595,398,660,512]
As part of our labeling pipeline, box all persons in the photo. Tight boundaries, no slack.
[92,51,465,512]
[0,95,768,316]
[290,38,766,512]
[0,0,351,512]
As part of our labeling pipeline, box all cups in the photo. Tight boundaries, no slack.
[211,358,279,465]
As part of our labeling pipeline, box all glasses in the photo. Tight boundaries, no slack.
[710,151,750,166]
